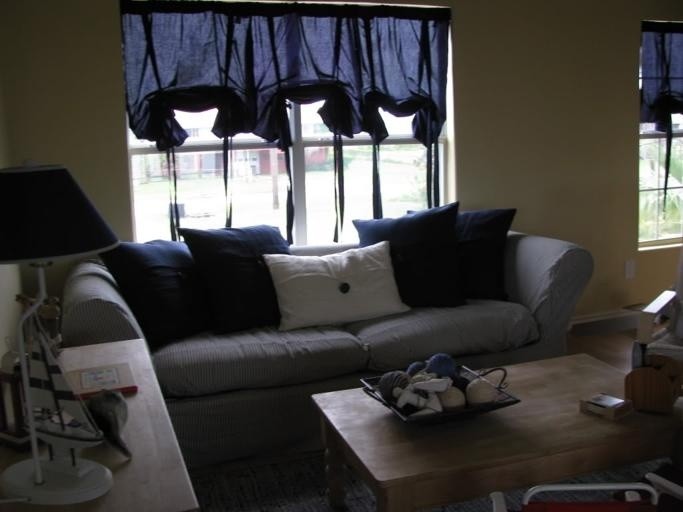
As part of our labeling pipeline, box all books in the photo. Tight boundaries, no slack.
[63,362,137,402]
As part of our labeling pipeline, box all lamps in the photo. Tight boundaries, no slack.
[0,164,120,506]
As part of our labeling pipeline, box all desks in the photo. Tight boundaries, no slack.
[0,338,201,512]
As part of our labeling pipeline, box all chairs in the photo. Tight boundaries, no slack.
[631,257,683,369]
[614,437,683,512]
[490,483,662,511]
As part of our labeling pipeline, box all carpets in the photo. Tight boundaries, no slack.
[187,449,675,511]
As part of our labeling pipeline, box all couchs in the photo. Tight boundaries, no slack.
[64,229,594,470]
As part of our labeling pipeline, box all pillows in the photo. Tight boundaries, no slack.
[119,239,208,351]
[262,240,411,332]
[352,202,460,306]
[447,208,517,304]
[175,226,292,336]
